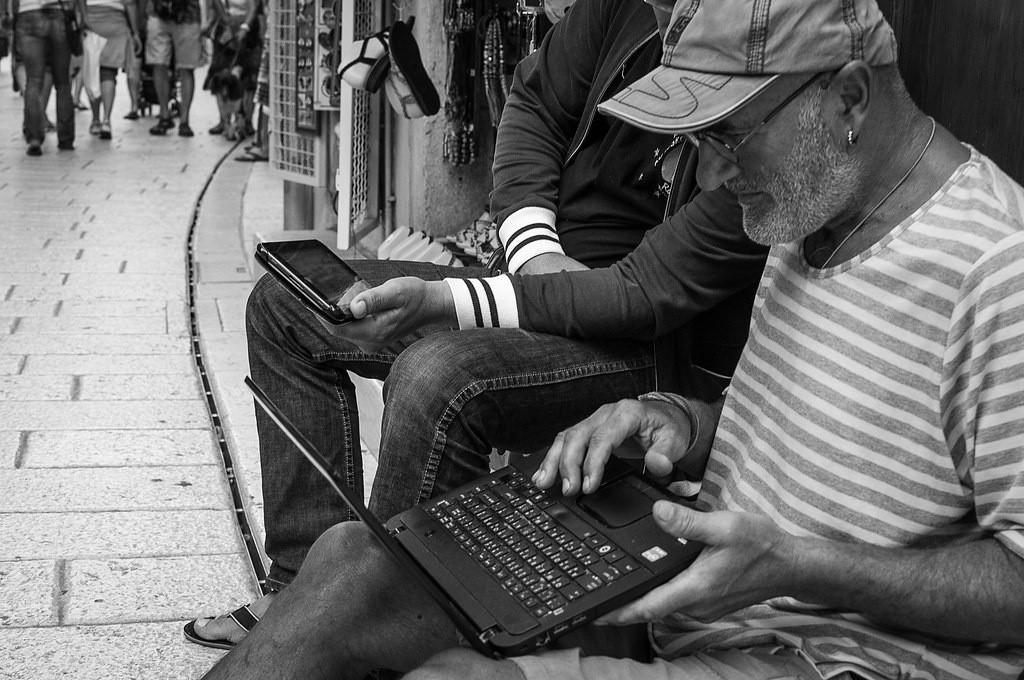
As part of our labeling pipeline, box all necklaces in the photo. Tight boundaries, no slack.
[816,116,936,277]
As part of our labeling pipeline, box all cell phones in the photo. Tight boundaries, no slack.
[253,240,373,326]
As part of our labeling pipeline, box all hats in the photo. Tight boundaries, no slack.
[597,0,899,135]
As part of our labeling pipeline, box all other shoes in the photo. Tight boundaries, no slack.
[26,140,43,156]
[149,115,176,136]
[59,143,75,150]
[90,121,112,140]
[178,122,194,137]
[209,122,269,162]
[124,109,138,120]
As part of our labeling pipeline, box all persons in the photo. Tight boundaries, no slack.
[181,0,1023,679]
[0,1,270,162]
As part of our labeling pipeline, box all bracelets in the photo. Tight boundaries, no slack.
[638,390,700,456]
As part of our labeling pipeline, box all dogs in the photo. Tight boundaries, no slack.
[211,66,258,142]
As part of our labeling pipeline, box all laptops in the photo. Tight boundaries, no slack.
[244,374,712,661]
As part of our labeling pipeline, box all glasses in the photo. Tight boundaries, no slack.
[685,69,833,164]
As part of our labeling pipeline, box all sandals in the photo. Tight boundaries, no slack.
[385,20,441,120]
[339,14,416,93]
[183,602,260,650]
